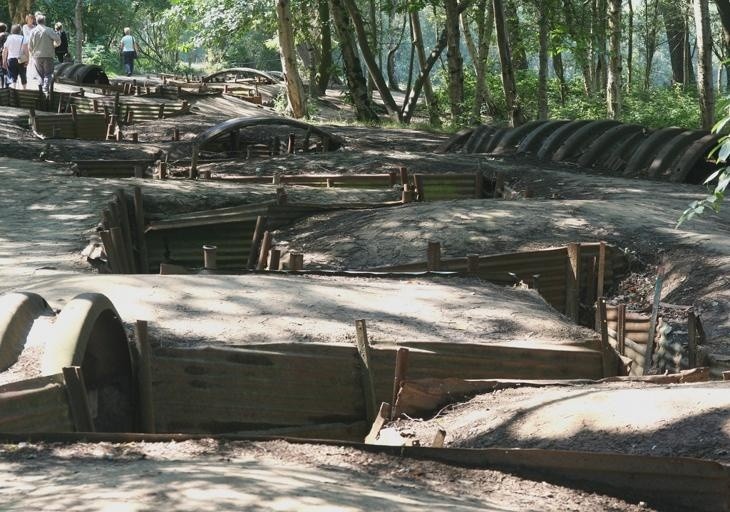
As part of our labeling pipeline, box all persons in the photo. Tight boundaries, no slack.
[2,23,28,89]
[28,13,64,103]
[22,14,40,82]
[51,22,69,63]
[117,26,140,76]
[0,21,10,90]
[34,11,44,20]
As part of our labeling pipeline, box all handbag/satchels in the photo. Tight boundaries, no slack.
[18,54,28,64]
[132,51,137,58]
